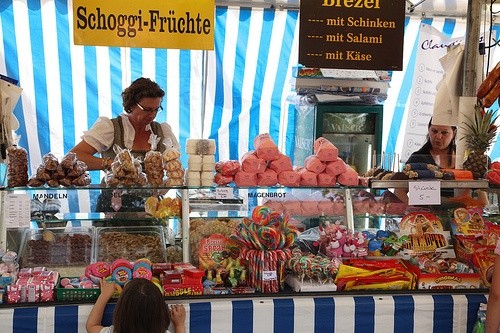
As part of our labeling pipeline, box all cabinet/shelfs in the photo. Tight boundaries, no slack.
[294,103,384,230]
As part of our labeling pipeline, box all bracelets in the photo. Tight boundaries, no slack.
[101,158,108,173]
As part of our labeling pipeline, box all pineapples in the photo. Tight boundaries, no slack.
[458,105,500,179]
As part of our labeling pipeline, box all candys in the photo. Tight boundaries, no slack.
[231,205,297,263]
[288,248,342,285]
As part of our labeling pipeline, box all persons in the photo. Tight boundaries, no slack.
[86,277,186,333]
[69,79,181,245]
[394,117,491,205]
[487,238,500,333]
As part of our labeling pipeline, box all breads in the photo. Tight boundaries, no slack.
[5,148,184,188]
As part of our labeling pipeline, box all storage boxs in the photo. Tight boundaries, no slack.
[55,276,101,303]
[94,226,168,262]
[335,259,414,290]
[163,270,181,278]
[39,271,59,302]
[17,226,96,267]
[181,276,202,284]
[26,276,40,302]
[284,273,337,293]
[183,269,205,278]
[173,263,196,274]
[162,283,204,296]
[0,268,20,289]
[160,274,182,285]
[0,288,6,304]
[189,198,249,212]
[7,276,30,303]
[401,258,482,289]
[151,262,173,270]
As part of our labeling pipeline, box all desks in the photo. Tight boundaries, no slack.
[0,290,491,333]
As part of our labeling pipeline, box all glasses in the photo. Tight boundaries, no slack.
[136,102,163,114]
